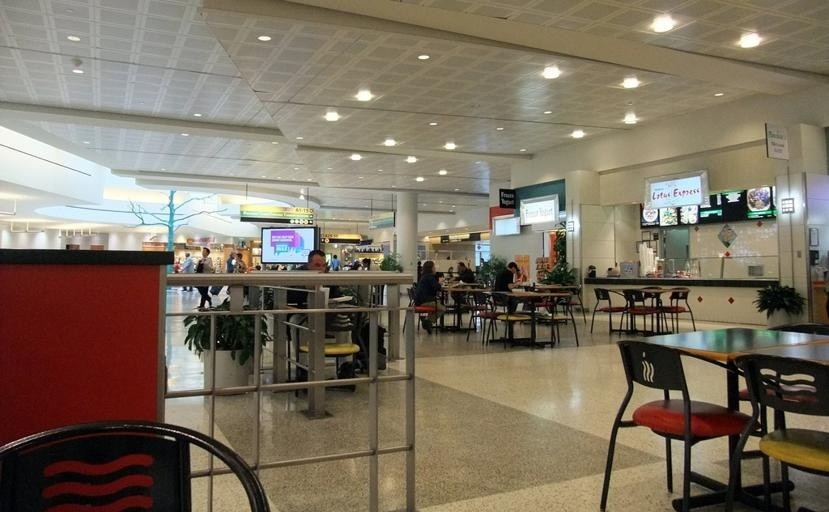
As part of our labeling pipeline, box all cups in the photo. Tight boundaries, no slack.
[639,242,659,277]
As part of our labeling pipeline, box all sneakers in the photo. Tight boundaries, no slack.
[420,316,426,330]
[424,320,433,335]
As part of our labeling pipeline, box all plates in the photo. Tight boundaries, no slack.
[643,208,659,223]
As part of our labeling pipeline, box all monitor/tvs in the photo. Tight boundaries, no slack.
[261,226,319,264]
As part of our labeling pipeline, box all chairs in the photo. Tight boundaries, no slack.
[402,285,697,346]
[295,302,368,397]
[0,420,271,511]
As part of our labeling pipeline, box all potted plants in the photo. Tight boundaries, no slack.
[752,282,810,328]
[182,297,273,397]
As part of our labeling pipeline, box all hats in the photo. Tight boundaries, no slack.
[509,263,521,272]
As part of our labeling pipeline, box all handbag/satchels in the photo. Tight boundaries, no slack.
[410,282,417,299]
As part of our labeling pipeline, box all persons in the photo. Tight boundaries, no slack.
[175,261,181,273]
[255,265,261,271]
[413,259,475,335]
[225,250,237,294]
[282,249,359,395]
[329,254,341,272]
[192,247,215,309]
[354,261,363,271]
[361,258,385,305]
[232,253,247,274]
[491,262,523,316]
[178,252,195,291]
